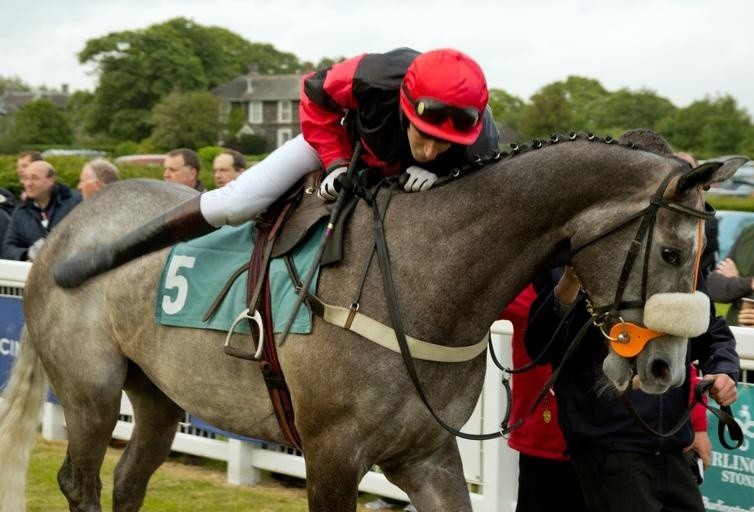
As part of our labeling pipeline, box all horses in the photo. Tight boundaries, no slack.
[0,129,754,512]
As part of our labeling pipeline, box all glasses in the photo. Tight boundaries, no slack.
[401,79,483,131]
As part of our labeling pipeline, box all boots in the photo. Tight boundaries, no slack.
[53,193,221,289]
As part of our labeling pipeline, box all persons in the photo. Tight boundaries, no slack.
[525,265,740,509]
[0,150,248,262]
[54,47,501,290]
[495,282,713,511]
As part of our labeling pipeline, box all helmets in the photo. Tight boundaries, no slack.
[399,48,489,146]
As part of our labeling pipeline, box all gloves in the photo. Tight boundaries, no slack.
[398,166,438,192]
[316,166,348,201]
